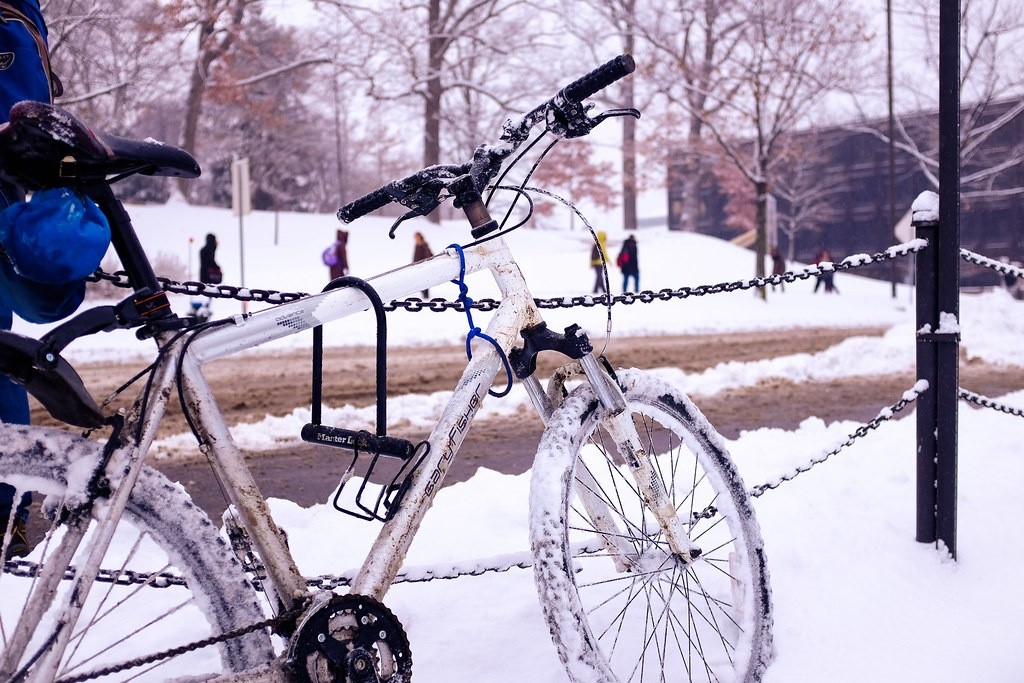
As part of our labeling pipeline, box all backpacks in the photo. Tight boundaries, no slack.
[322,243,342,268]
[592,242,599,260]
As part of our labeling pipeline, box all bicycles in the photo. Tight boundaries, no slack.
[0,54,775,681]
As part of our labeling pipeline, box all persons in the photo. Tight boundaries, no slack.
[771,248,785,291]
[200,234,218,285]
[809,249,836,292]
[413,233,433,299]
[331,230,348,279]
[617,235,639,293]
[0,0,52,559]
[590,231,613,294]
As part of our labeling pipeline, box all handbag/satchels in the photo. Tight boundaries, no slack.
[617,256,621,267]
